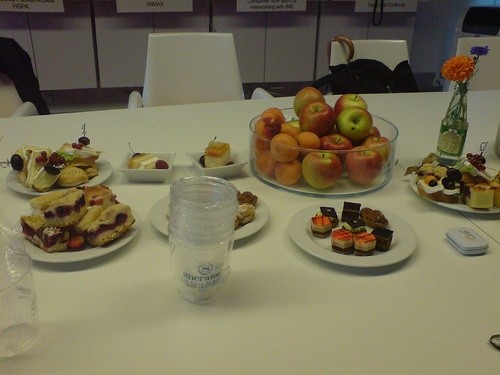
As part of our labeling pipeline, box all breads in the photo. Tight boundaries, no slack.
[233,189,259,229]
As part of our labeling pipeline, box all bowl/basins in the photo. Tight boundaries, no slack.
[187,150,248,178]
[118,151,176,182]
[246,107,399,196]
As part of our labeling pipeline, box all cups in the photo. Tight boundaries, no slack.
[167,175,239,305]
[0,252,41,360]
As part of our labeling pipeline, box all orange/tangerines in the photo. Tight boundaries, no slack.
[253,108,322,186]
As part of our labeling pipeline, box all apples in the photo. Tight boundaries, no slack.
[289,86,390,189]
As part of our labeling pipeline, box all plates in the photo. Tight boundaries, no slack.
[8,155,113,195]
[12,202,138,263]
[410,166,500,213]
[288,201,418,268]
[148,192,269,241]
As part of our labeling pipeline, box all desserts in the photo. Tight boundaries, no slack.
[311,201,394,257]
[10,135,101,192]
[198,136,233,168]
[403,152,500,209]
[128,142,169,169]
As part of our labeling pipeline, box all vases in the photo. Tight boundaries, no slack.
[437,82,470,160]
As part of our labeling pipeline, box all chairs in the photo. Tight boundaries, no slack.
[327,39,411,95]
[446,36,500,92]
[128,32,272,110]
[0,37,41,117]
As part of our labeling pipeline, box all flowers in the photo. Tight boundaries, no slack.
[440,45,491,158]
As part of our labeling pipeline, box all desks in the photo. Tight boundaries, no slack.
[0,89,500,375]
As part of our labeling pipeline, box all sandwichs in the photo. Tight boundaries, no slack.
[19,184,136,252]
